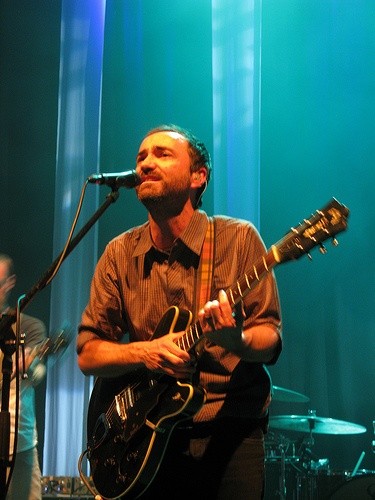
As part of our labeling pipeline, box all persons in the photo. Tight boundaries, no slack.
[0,254,46,500]
[76,124,283,500]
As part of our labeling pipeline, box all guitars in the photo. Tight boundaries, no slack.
[86,195,352,500]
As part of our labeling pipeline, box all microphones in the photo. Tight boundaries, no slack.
[87,168,144,190]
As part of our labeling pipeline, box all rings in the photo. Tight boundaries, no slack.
[205,317,210,320]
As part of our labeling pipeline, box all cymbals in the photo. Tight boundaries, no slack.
[268,414,368,436]
[270,384,312,404]
